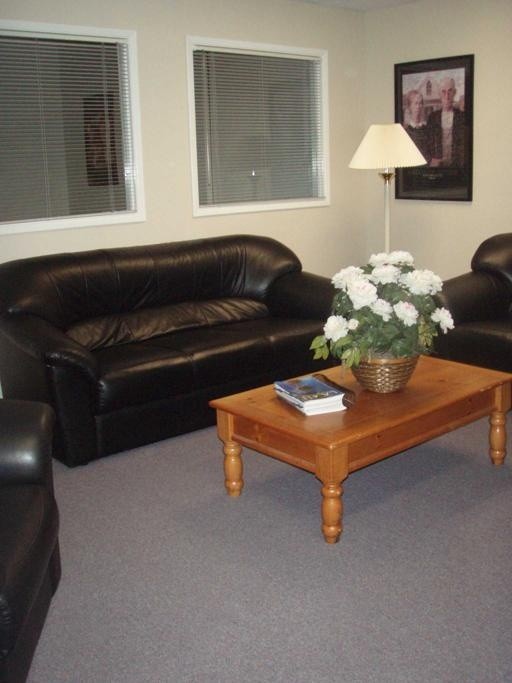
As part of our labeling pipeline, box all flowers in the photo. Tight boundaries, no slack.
[308,252,454,368]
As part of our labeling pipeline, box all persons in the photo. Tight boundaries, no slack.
[402,91,432,167]
[427,79,467,170]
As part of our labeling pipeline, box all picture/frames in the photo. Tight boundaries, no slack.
[393,55,474,202]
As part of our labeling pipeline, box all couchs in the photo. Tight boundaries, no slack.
[0,233,338,468]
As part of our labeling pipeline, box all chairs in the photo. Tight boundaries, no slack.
[0,399,64,683]
[431,233,512,375]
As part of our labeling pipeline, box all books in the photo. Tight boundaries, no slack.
[274,376,348,416]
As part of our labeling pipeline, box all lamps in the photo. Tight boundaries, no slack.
[348,123,427,252]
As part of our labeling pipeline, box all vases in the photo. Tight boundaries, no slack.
[350,358,420,394]
